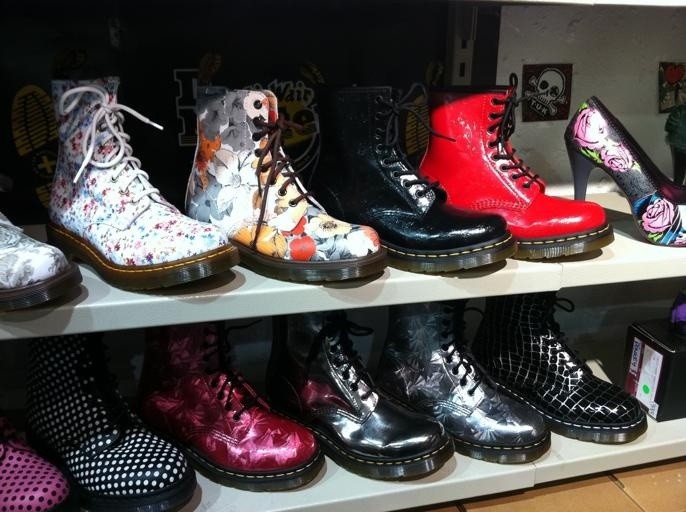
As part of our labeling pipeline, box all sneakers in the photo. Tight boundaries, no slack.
[1,407,81,511]
[0,210,84,314]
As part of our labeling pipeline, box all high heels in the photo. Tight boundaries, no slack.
[563,96,685,247]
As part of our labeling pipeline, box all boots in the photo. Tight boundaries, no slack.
[45,74,242,292]
[374,299,552,464]
[310,84,516,275]
[470,291,646,444]
[184,86,386,282]
[416,83,614,259]
[20,334,199,511]
[135,320,326,492]
[261,309,453,478]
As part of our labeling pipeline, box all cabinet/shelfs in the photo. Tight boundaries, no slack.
[1,192,686,511]
